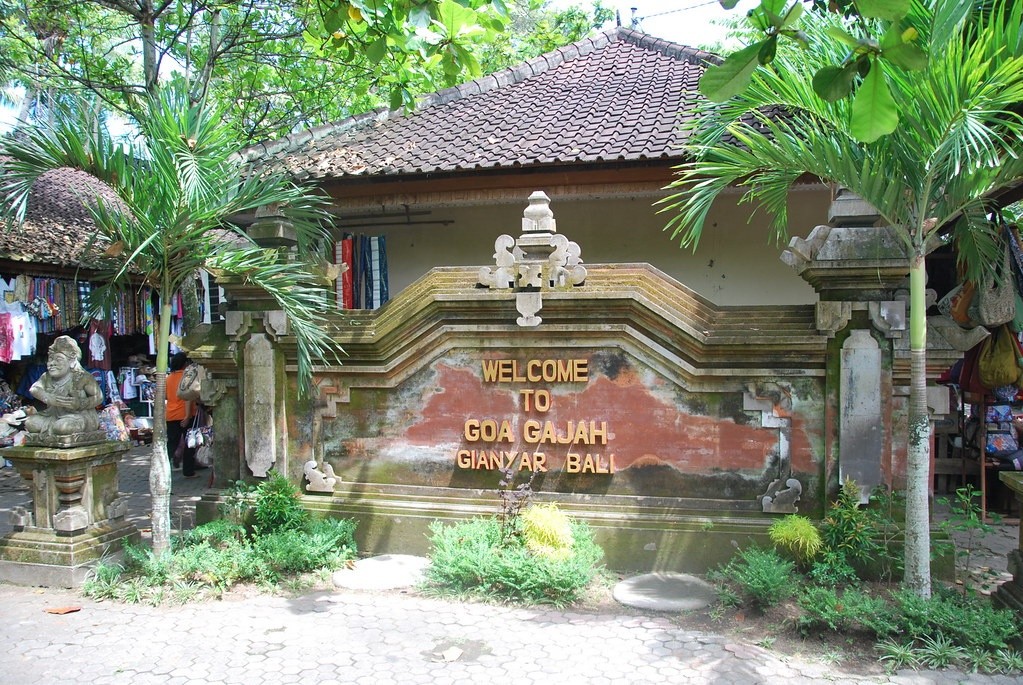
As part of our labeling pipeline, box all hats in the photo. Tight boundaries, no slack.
[0,408,37,445]
[129,353,157,383]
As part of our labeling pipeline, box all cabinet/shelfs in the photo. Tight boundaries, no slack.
[121,367,153,418]
[959,392,1023,526]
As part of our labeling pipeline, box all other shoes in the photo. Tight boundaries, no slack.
[172,455,179,469]
[182,473,200,479]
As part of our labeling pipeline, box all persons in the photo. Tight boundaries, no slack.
[165,353,199,476]
[24,335,103,434]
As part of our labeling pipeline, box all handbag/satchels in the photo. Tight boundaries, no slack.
[939,208,1023,472]
[176,365,202,401]
[185,409,214,468]
